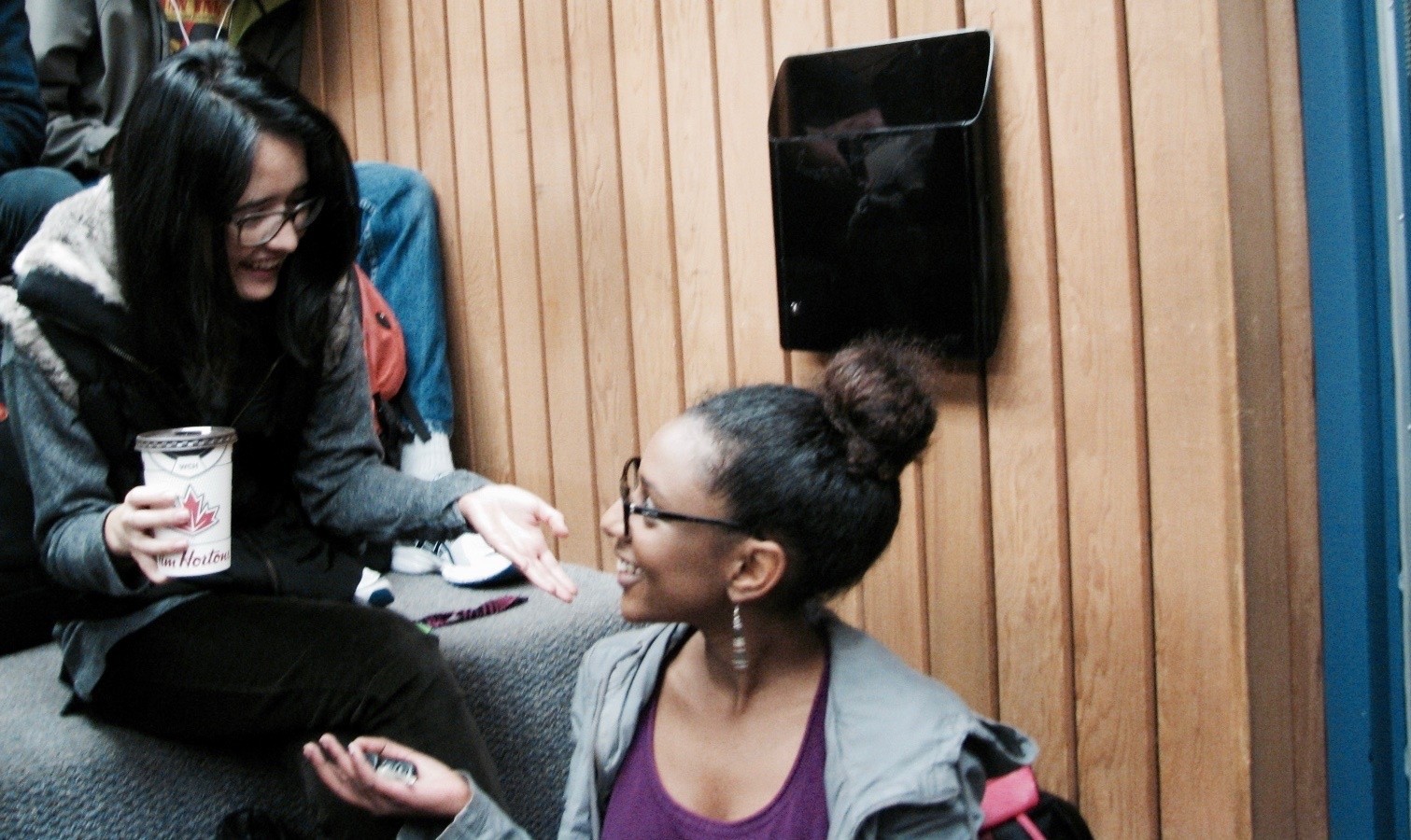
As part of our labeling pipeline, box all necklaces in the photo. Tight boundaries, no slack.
[171,1,231,50]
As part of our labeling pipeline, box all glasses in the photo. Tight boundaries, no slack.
[619,456,768,542]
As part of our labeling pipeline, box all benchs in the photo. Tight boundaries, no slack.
[0,549,647,840]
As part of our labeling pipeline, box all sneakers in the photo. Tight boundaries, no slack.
[390,528,515,586]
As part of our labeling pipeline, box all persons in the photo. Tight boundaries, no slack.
[0,1,81,268]
[22,0,525,607]
[305,349,1042,840]
[0,39,577,839]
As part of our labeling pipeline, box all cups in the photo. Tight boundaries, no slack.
[135,426,232,577]
